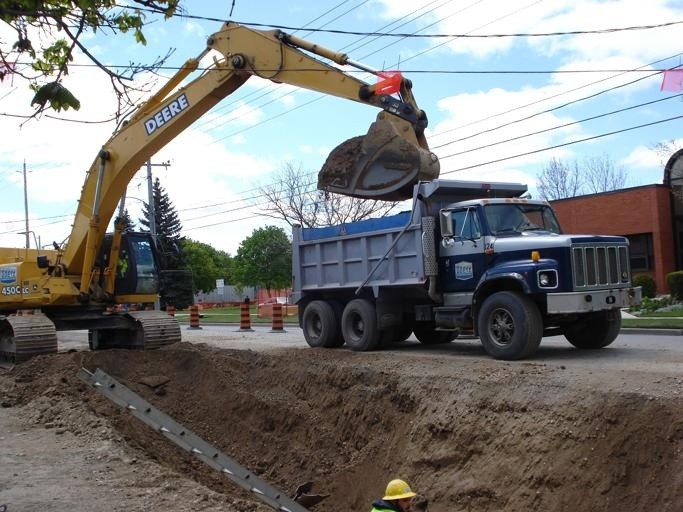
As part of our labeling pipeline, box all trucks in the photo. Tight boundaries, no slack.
[285,173,644,359]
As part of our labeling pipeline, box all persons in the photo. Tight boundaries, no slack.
[118,249,128,277]
[370,479,419,512]
[245,295,250,305]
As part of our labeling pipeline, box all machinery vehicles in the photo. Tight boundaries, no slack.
[0,21,440,358]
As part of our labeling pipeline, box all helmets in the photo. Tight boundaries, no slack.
[381,478,416,501]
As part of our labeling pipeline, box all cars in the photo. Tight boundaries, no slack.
[259,296,287,309]
